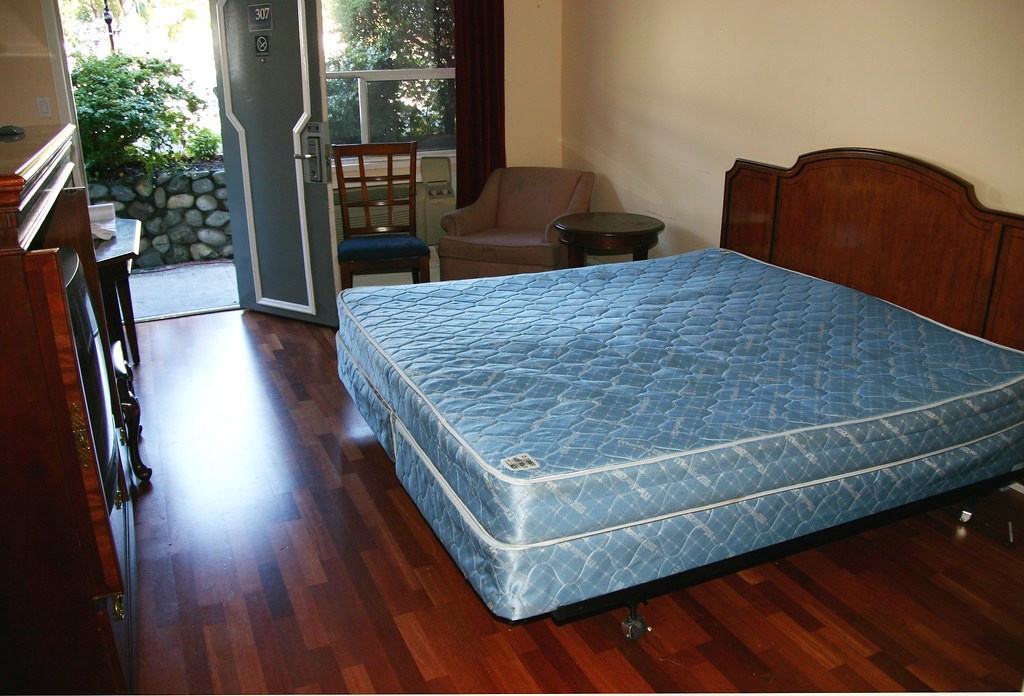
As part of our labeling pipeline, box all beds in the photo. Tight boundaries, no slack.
[334,146,1024,629]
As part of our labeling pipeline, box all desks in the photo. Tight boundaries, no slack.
[93,218,142,365]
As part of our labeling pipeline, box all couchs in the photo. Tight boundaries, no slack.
[438,167,596,283]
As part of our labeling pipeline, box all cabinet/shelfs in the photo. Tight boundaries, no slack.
[0,124,136,695]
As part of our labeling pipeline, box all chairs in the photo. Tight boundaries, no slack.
[332,140,432,290]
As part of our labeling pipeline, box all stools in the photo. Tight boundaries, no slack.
[111,340,153,483]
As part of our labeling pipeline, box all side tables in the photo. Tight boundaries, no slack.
[553,212,665,269]
